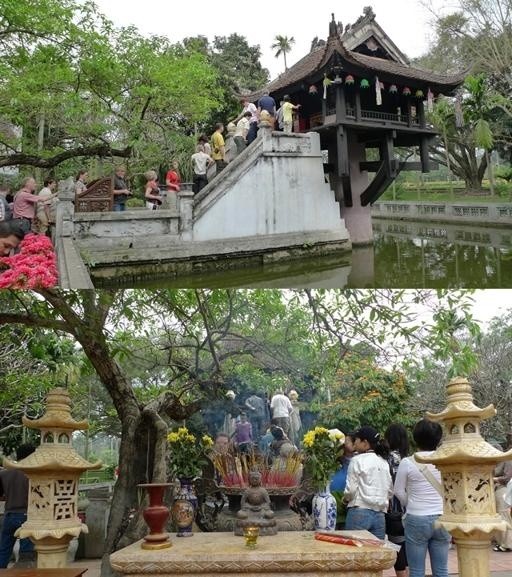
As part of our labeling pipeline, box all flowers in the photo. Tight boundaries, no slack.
[163,424,215,484]
[300,425,346,493]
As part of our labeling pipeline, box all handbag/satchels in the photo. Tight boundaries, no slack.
[385,511,405,537]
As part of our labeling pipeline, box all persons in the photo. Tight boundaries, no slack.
[393,418,452,577]
[492,432,512,553]
[1,444,36,570]
[1,87,302,271]
[236,467,277,534]
[211,388,409,577]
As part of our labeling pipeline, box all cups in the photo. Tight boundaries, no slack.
[243,526,258,548]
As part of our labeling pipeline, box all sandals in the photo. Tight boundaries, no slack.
[493,546,510,552]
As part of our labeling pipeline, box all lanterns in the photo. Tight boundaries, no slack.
[322,74,332,88]
[402,87,411,96]
[308,85,318,95]
[359,78,370,90]
[415,89,425,98]
[379,81,384,91]
[344,74,354,86]
[389,83,397,94]
[334,74,342,84]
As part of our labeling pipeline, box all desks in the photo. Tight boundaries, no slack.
[107,530,397,577]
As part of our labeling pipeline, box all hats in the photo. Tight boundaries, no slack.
[199,136,209,141]
[347,428,380,443]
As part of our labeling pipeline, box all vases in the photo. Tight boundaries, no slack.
[171,477,198,538]
[311,479,337,535]
[135,481,174,550]
